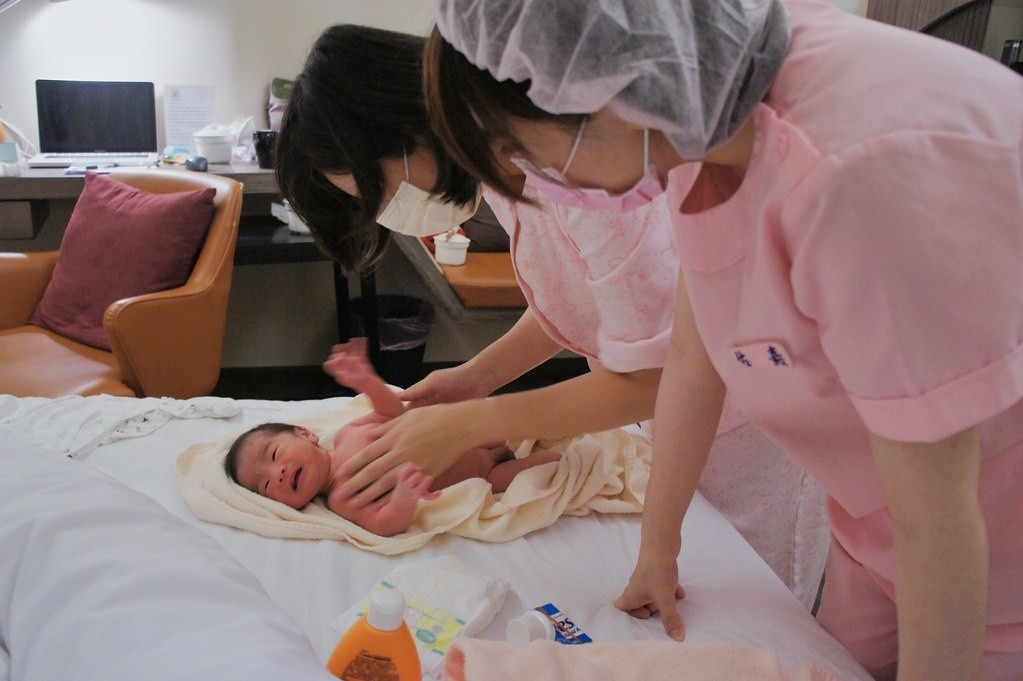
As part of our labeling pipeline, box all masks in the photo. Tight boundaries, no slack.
[509,117,669,214]
[375,150,482,238]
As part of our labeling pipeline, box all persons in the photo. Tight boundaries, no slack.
[421,1,1023,681]
[272,24,829,617]
[224,335,562,537]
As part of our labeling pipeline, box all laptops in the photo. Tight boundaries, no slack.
[27,81,166,168]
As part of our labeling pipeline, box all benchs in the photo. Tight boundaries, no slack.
[390,229,589,362]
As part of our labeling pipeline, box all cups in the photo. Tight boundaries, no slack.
[253,130,278,169]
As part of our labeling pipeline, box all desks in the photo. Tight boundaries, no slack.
[0,163,380,363]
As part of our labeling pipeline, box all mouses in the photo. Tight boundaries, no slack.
[186,157,208,172]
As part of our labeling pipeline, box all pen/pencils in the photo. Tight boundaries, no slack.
[86,164,118,170]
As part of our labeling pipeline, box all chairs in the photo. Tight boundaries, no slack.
[0,168,244,398]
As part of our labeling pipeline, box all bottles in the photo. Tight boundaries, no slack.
[326,589,422,680]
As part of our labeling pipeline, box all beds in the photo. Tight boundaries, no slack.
[0,394,881,680]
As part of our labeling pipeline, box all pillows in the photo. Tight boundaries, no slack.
[27,172,217,351]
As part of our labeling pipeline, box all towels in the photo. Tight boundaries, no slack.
[443,632,838,681]
[170,382,654,557]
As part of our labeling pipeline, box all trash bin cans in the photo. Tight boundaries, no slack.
[1000,40,1022,66]
[348,295,435,378]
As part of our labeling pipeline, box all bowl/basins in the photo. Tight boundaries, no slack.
[192,132,233,164]
[433,233,470,265]
